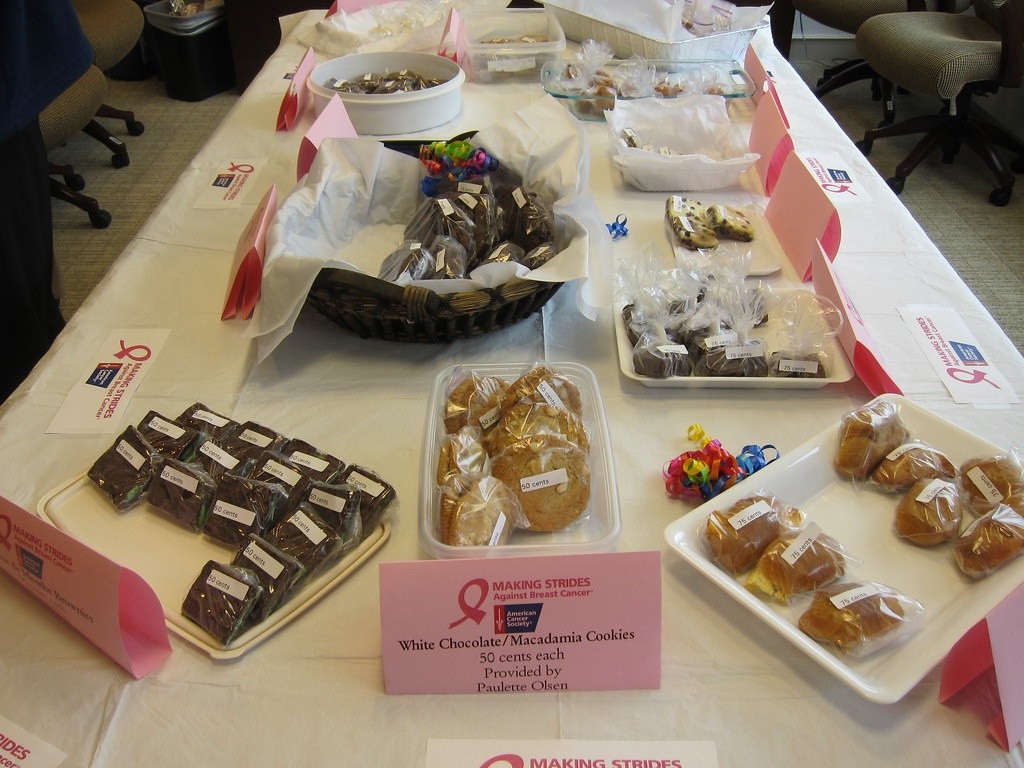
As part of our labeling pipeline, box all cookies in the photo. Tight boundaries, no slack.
[436,367,590,547]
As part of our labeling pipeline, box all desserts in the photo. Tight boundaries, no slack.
[87,402,396,646]
[336,34,723,279]
[622,276,826,378]
[666,194,752,250]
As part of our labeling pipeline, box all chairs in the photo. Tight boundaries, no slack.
[38,63,114,230]
[71,0,145,168]
[853,0,1024,206]
[791,0,973,130]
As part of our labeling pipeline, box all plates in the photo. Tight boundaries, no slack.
[666,205,781,277]
[35,468,392,659]
[602,117,753,190]
[664,390,1023,705]
[540,60,756,121]
[612,289,856,391]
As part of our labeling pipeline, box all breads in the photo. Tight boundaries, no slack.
[706,401,1022,647]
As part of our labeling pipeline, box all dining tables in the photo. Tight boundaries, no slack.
[1,9,1024,768]
